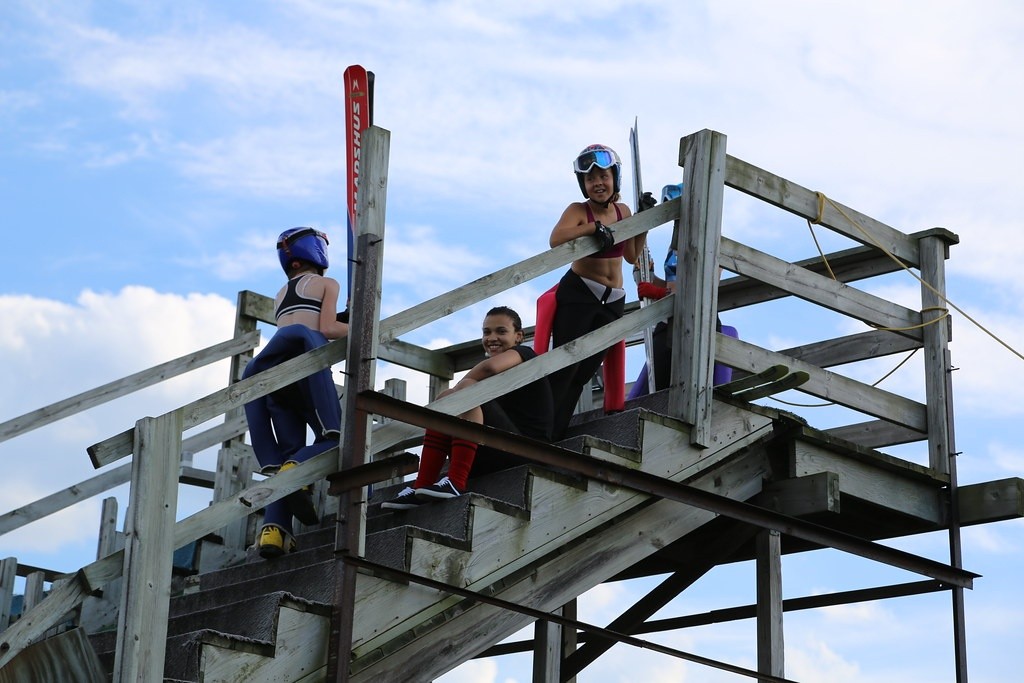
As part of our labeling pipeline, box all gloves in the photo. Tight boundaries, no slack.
[593,222,614,249]
[638,282,670,300]
[637,192,657,213]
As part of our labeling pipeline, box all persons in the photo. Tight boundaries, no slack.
[380,306,554,511]
[631,181,723,392]
[240,226,349,560]
[549,144,657,444]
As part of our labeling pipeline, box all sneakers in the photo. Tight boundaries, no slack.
[380,486,425,508]
[278,460,320,526]
[258,523,298,559]
[415,476,466,499]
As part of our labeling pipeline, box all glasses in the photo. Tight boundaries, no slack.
[572,149,620,173]
[661,185,681,203]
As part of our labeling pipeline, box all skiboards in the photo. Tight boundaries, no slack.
[628,115,658,392]
[713,365,810,404]
[343,65,374,311]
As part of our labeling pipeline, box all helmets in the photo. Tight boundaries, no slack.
[276,227,330,273]
[573,144,621,200]
